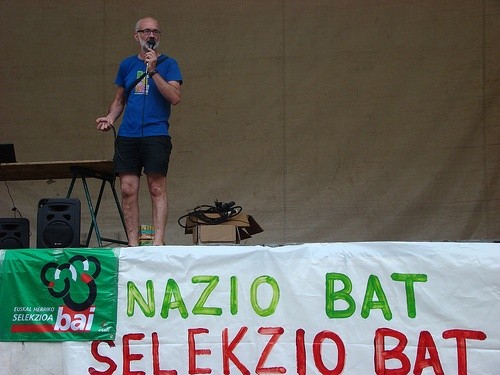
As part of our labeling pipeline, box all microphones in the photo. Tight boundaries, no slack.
[145,39,157,68]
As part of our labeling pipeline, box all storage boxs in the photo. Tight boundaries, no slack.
[193,225,241,246]
[184,212,264,239]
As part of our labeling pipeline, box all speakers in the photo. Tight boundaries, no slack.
[0,217,30,249]
[36,197,81,249]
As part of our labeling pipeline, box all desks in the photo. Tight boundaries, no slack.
[0,160,130,248]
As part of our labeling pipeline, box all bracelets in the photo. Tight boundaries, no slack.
[149,70,158,79]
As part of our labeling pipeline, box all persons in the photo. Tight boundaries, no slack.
[96,18,183,247]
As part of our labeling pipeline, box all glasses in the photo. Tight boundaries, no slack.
[136,29,161,36]
